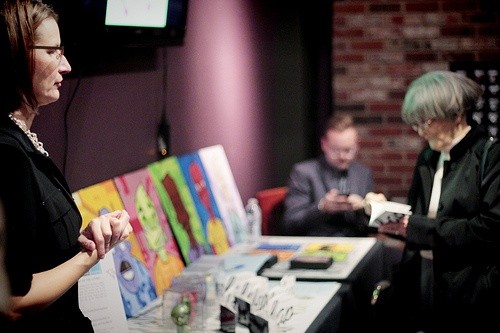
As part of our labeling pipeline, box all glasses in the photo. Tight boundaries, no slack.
[413,117,435,131]
[31,44,65,55]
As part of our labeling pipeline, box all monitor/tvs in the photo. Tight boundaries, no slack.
[105,0,189,47]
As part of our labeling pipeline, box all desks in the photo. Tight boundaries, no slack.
[228,235,384,333]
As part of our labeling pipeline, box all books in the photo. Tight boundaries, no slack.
[78,243,162,333]
[368,198,412,228]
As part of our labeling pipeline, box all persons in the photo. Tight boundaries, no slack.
[280,112,375,236]
[0,0,133,333]
[364,71,500,333]
